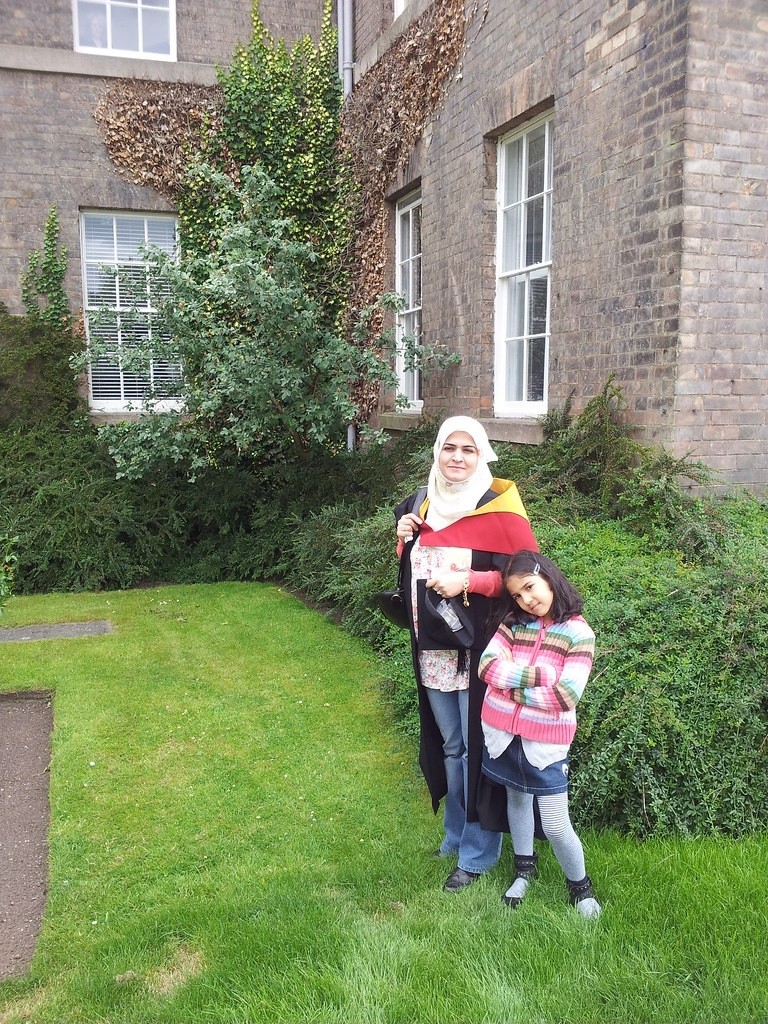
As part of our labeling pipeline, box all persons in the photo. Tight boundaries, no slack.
[477,550,602,919]
[393,415,539,893]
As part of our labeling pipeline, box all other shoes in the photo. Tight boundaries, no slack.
[444,868,480,892]
[432,849,444,857]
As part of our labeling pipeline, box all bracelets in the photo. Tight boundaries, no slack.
[462,572,469,607]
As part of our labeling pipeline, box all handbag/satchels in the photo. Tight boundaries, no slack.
[378,587,412,630]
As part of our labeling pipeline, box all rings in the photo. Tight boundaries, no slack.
[403,526,405,530]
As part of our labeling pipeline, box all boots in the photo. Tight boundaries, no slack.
[568,877,601,919]
[504,852,539,909]
